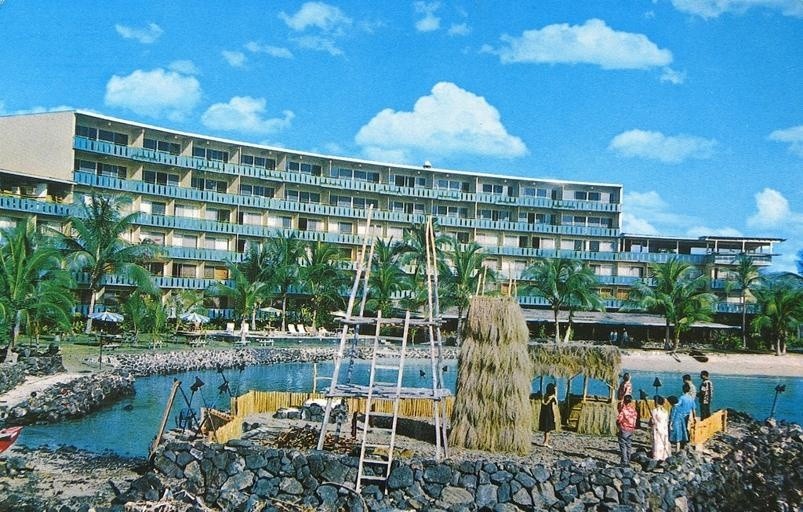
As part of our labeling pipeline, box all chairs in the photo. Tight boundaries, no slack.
[226,322,235,335]
[288,324,335,338]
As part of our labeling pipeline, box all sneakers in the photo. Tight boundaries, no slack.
[544,443,552,449]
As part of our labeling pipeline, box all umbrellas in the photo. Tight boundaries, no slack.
[86,311,124,323]
[177,312,210,324]
[329,309,346,317]
[259,306,281,313]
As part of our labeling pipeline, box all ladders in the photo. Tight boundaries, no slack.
[355,309,411,494]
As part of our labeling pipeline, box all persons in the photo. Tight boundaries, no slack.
[616,373,632,414]
[666,395,689,452]
[538,383,557,448]
[615,395,637,465]
[647,394,672,462]
[681,374,697,401]
[695,370,712,421]
[675,384,696,431]
